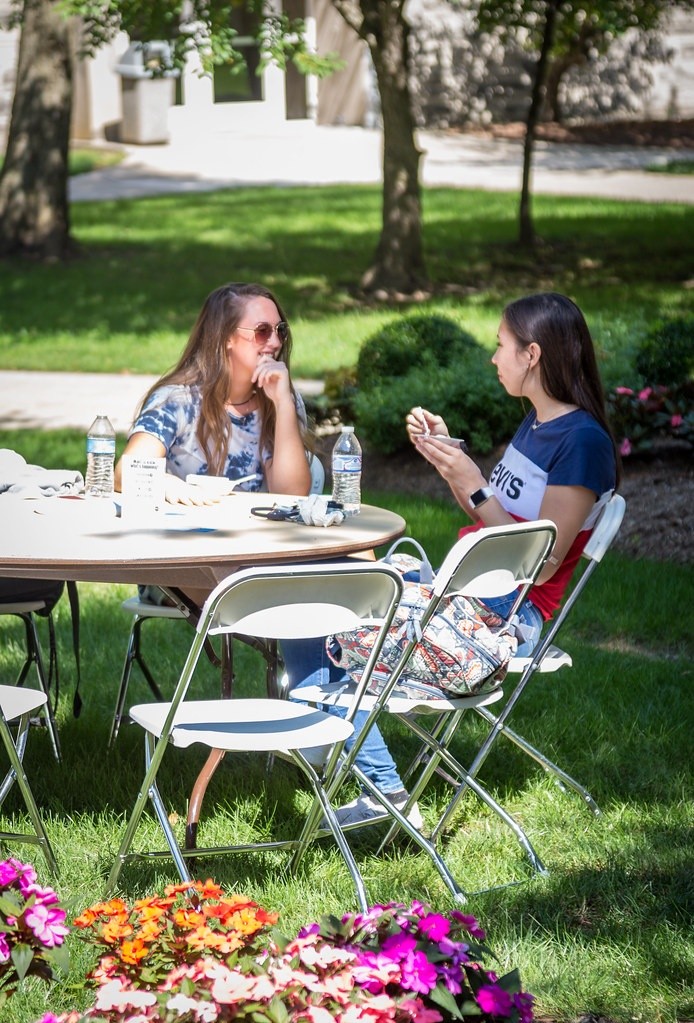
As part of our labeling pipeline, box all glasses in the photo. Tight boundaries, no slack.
[236,321,290,346]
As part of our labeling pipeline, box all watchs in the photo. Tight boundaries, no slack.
[468,487,496,511]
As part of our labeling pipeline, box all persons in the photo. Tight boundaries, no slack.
[113,282,376,606]
[270,291,622,831]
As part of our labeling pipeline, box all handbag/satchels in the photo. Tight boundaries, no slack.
[324,537,517,701]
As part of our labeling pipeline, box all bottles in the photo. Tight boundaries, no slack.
[331,426,362,518]
[85,411,116,510]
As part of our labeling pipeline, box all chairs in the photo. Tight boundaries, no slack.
[0,450,627,928]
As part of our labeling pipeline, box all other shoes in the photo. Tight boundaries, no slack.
[272,745,351,773]
[318,790,424,833]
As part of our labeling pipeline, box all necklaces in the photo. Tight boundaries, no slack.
[226,387,257,405]
[532,405,572,430]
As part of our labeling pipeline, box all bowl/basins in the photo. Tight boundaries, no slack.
[411,433,463,448]
[187,474,230,499]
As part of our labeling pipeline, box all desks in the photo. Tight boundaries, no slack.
[0,491,407,863]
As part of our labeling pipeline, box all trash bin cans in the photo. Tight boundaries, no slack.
[110,41,182,145]
